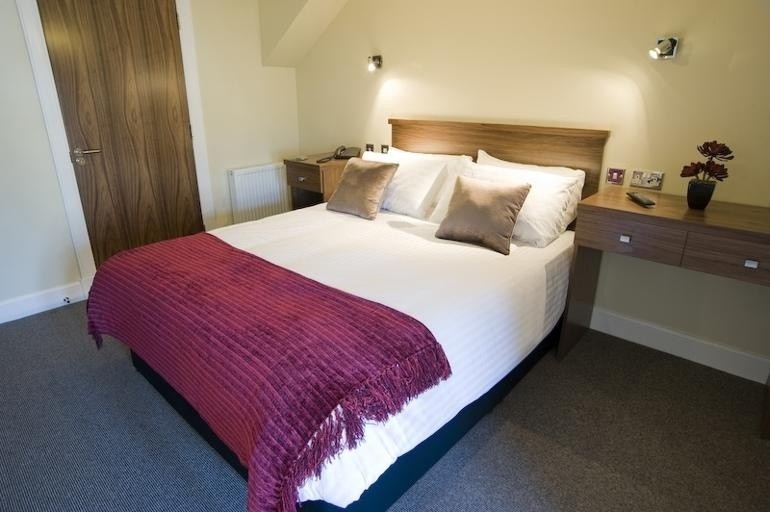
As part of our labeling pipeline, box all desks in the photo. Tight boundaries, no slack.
[555,182,770,361]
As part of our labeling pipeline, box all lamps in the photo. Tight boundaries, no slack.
[368,55,382,71]
[648,37,679,61]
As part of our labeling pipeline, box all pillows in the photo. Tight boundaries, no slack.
[326,146,586,256]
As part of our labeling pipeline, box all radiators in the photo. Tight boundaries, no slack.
[228,162,289,224]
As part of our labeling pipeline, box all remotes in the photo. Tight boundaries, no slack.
[626,191,656,208]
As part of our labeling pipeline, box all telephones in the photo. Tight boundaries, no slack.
[334,145,361,159]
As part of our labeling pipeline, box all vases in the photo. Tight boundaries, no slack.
[686,179,717,210]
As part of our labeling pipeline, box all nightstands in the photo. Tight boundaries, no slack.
[283,152,349,210]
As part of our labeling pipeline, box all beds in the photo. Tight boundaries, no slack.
[86,118,611,510]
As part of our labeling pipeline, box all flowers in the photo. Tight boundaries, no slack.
[680,139,734,184]
